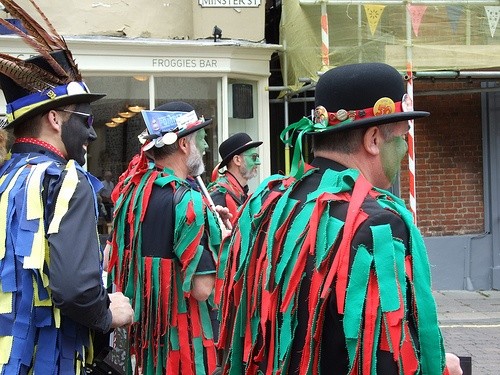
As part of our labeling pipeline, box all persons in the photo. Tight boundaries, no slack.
[0,0,135,375]
[215,62,462,375]
[103,101,264,375]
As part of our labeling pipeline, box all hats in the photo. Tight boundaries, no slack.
[210,132,264,181]
[280,62,432,181]
[137,102,214,152]
[0,0,107,128]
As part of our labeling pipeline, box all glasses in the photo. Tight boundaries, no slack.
[41,107,95,128]
